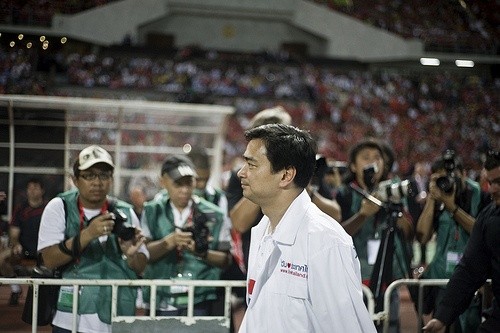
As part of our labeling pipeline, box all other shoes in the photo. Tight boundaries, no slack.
[10,293,21,305]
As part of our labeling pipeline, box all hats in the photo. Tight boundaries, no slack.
[74,145,115,175]
[162,156,199,181]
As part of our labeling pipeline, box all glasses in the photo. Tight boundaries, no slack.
[487,151,500,160]
[80,174,112,180]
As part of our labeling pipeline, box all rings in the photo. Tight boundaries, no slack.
[104,226,106,231]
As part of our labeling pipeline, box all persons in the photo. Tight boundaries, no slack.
[237,122,377,333]
[0,0,500,333]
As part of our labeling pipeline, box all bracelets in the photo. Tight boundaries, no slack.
[453,208,458,215]
[310,184,318,199]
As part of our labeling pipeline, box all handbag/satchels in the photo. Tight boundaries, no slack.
[22,268,61,326]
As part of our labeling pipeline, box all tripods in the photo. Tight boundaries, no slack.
[365,202,429,333]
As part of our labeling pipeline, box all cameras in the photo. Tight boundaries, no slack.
[436,145,456,193]
[182,211,209,253]
[363,162,419,205]
[105,204,135,240]
